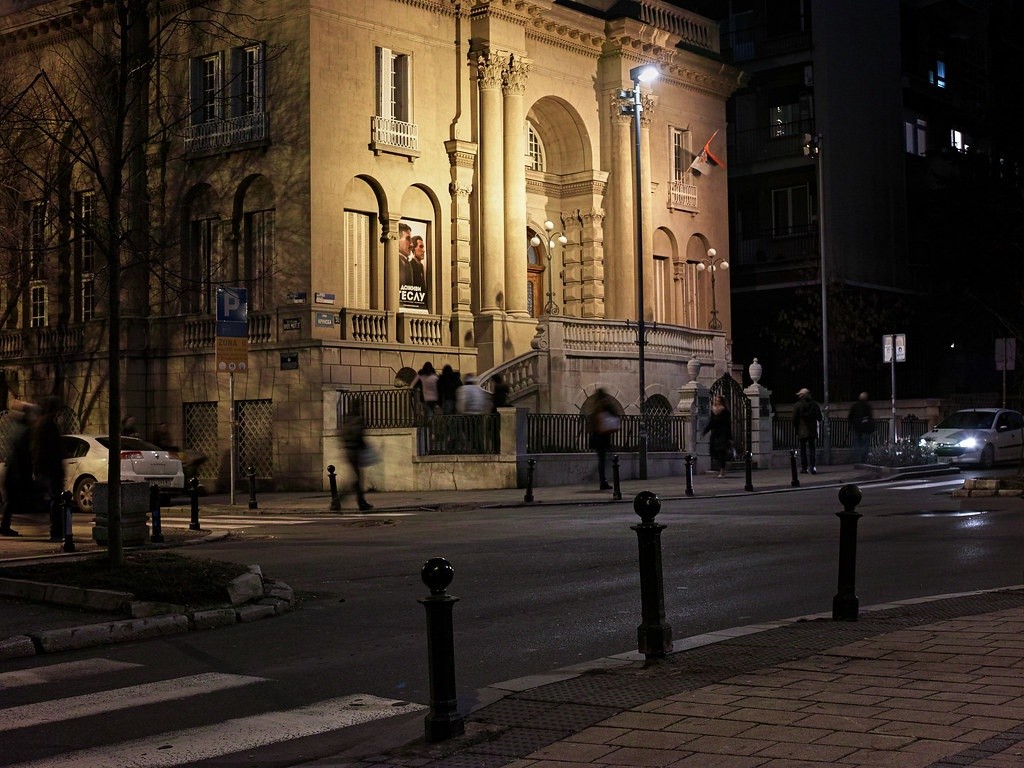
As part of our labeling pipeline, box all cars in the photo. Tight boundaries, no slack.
[58,434,185,514]
[917,409,1024,469]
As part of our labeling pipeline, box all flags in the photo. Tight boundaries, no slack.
[689,143,724,176]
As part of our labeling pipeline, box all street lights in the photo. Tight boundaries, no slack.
[697,248,730,329]
[530,221,568,316]
[616,63,658,477]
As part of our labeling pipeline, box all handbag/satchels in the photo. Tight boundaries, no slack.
[350,444,383,467]
[595,399,621,432]
[859,416,876,433]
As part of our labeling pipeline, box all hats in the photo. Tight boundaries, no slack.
[795,388,810,395]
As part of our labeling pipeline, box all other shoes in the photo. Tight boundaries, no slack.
[599,480,613,490]
[810,465,817,474]
[800,468,809,473]
[360,502,374,511]
[717,468,726,478]
[0,525,19,536]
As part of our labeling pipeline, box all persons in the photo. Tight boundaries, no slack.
[0,397,66,541]
[847,392,873,462]
[703,395,733,478]
[399,223,426,302]
[410,362,509,454]
[331,400,373,512]
[586,386,619,489]
[122,415,137,437]
[155,422,170,448]
[791,389,823,474]
